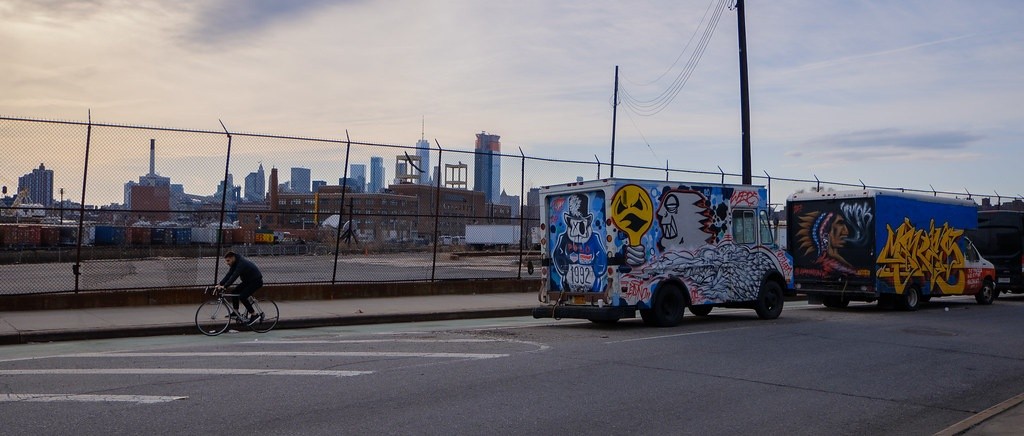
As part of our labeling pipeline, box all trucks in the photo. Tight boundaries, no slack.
[531,177,802,328]
[785,184,998,312]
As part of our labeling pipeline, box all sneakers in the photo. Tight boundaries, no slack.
[225,312,240,318]
[248,313,261,326]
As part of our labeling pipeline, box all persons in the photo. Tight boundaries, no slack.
[215,251,263,326]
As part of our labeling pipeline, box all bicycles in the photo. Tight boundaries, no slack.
[195,286,280,337]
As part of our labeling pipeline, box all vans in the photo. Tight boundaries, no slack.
[970,209,1024,299]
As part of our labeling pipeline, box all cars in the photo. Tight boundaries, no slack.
[358,233,466,247]
